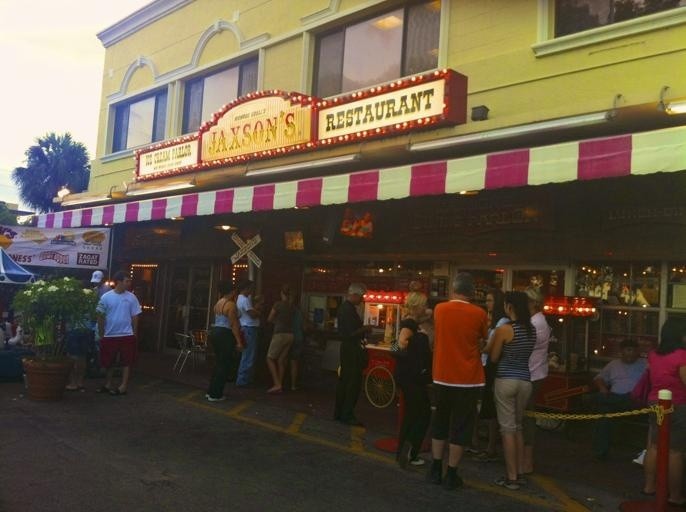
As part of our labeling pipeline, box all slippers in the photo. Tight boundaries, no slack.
[96,387,108,392]
[109,389,127,395]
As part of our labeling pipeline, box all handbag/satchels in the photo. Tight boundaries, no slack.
[412,352,433,385]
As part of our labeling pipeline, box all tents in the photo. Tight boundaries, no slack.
[0,246,39,284]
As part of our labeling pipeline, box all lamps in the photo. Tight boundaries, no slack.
[399,93,622,154]
[122,172,200,200]
[655,83,685,117]
[242,143,368,181]
[58,183,118,209]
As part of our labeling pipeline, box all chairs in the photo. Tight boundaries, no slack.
[191,328,211,367]
[170,327,205,374]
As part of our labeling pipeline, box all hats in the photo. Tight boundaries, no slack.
[90,271,104,282]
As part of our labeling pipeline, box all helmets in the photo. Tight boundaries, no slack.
[349,282,367,297]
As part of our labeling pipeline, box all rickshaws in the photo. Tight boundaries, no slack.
[355,289,406,411]
[527,293,598,432]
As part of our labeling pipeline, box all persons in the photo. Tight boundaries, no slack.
[91,270,142,394]
[1,274,94,347]
[594,314,686,507]
[334,283,373,427]
[204,278,305,400]
[397,269,550,490]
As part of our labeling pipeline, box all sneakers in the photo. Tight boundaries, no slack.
[632,449,647,464]
[408,448,463,490]
[468,448,534,490]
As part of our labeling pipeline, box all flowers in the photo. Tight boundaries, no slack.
[5,276,106,361]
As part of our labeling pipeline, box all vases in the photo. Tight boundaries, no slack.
[21,354,73,398]
